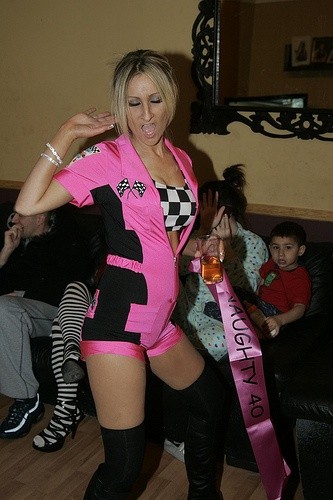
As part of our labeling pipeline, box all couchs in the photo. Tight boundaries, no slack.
[0,202,333,500]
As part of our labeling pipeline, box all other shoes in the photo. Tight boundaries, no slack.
[164,437,185,462]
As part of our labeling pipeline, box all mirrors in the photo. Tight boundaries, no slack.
[186,0,333,143]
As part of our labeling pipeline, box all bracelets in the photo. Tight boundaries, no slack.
[40,152,60,168]
[44,141,64,165]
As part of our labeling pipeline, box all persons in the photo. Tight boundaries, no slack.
[32,227,108,452]
[230,219,313,340]
[171,164,270,362]
[14,48,229,500]
[0,210,81,440]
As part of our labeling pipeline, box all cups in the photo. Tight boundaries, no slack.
[197,234,224,285]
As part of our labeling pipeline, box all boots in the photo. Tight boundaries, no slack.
[83,417,163,500]
[165,361,226,500]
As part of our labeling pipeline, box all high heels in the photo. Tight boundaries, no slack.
[59,340,87,394]
[32,398,84,452]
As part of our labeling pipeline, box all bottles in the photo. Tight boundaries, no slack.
[243,301,279,338]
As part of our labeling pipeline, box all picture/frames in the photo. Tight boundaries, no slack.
[290,35,333,68]
[224,92,308,108]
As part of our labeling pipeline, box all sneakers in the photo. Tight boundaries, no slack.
[0,393,45,440]
